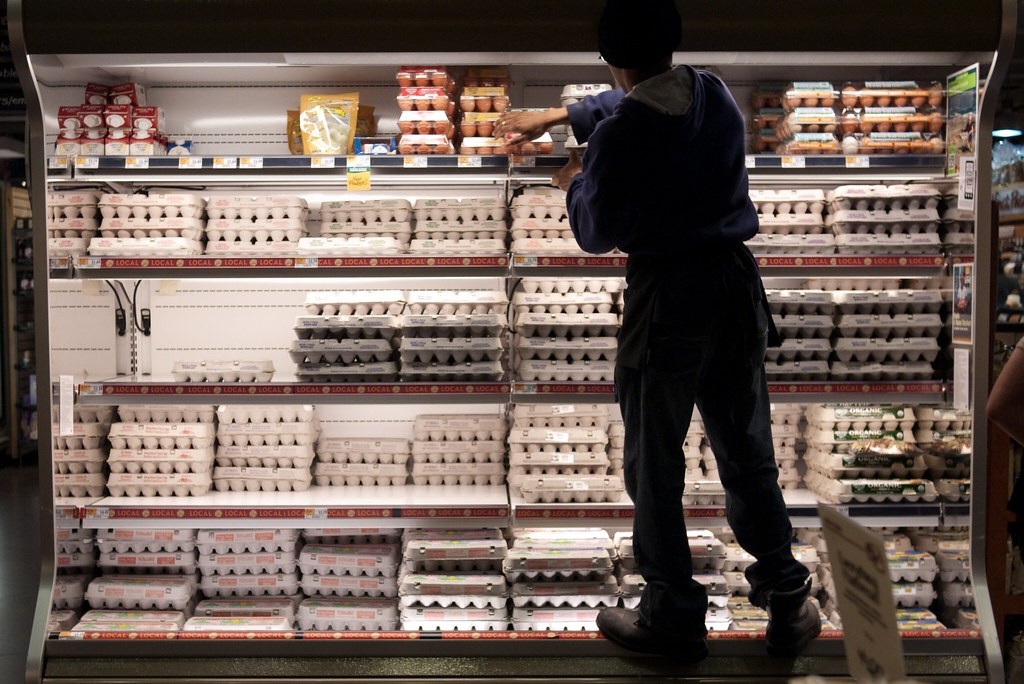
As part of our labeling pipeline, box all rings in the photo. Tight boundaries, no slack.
[502,121,505,126]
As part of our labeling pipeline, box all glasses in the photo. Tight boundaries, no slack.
[599,55,606,63]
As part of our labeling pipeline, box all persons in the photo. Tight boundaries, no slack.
[489,9,823,663]
[985,335,1024,564]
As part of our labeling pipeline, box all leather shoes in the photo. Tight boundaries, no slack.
[764,599,822,657]
[597,608,709,660]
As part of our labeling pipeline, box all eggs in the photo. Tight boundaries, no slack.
[46,71,1023,633]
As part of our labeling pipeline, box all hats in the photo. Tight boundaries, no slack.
[598,0,683,69]
